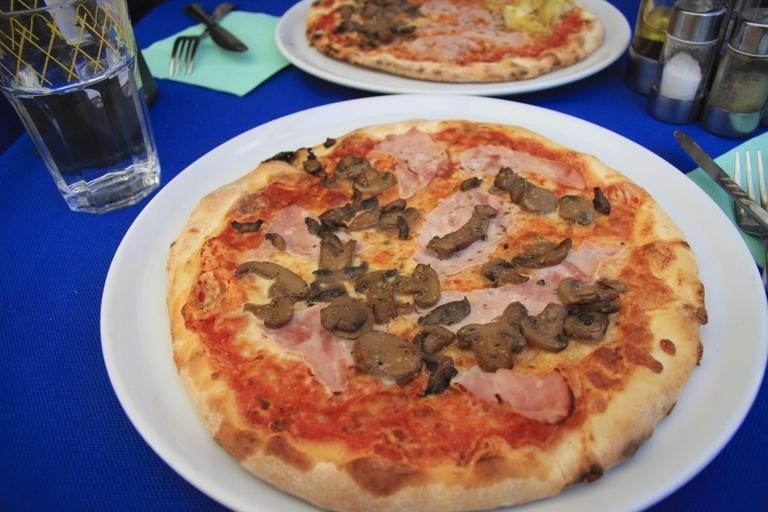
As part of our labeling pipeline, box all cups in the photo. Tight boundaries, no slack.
[0,0,162,215]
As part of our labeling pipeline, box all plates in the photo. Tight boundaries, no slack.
[274,0,634,96]
[99,94,768,511]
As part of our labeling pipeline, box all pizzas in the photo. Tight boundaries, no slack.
[169,119,706,512]
[306,0,605,83]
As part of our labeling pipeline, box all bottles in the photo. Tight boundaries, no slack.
[627,0,768,140]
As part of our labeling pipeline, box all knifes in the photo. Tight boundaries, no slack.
[671,129,768,232]
[184,4,248,53]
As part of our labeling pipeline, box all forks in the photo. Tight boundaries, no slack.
[169,3,238,77]
[733,148,768,240]
